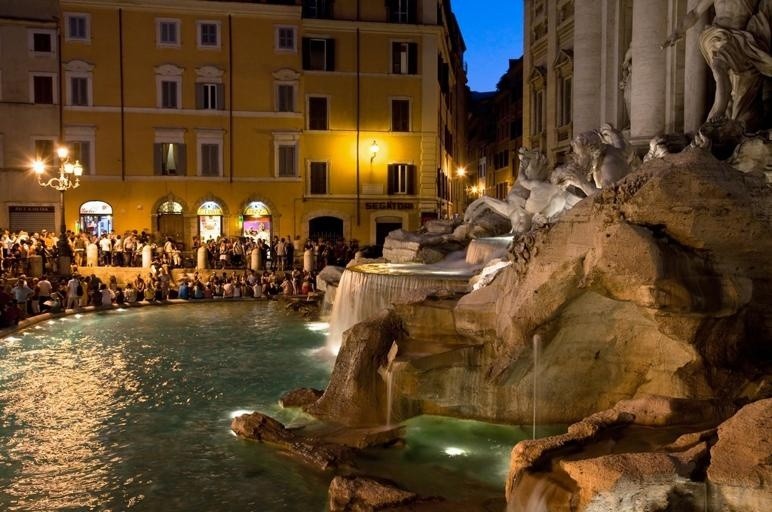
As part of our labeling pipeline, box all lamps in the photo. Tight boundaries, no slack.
[370,139,379,163]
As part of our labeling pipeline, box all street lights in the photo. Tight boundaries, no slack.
[34,145,84,276]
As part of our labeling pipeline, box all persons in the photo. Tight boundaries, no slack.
[257,228,269,241]
[249,226,257,234]
[1,228,183,327]
[176,267,319,300]
[457,109,772,237]
[87,221,94,234]
[191,234,368,272]
[659,0,771,125]
